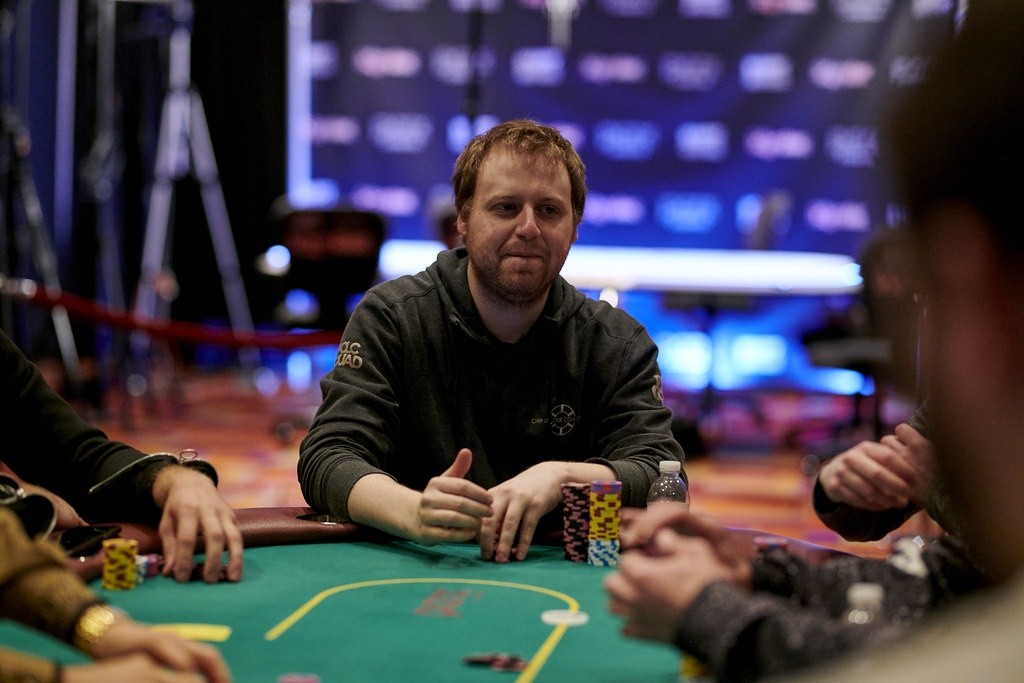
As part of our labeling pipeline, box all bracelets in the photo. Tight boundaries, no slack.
[76,604,112,649]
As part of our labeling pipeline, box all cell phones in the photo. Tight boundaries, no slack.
[60,524,122,555]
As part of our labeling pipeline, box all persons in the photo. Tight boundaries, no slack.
[0,327,242,683]
[296,120,689,563]
[603,0,1024,682]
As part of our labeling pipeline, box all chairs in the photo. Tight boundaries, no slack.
[262,206,395,441]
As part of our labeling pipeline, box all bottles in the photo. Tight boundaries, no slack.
[646,460,691,512]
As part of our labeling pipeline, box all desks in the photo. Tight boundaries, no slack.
[374,237,859,453]
[0,506,690,682]
